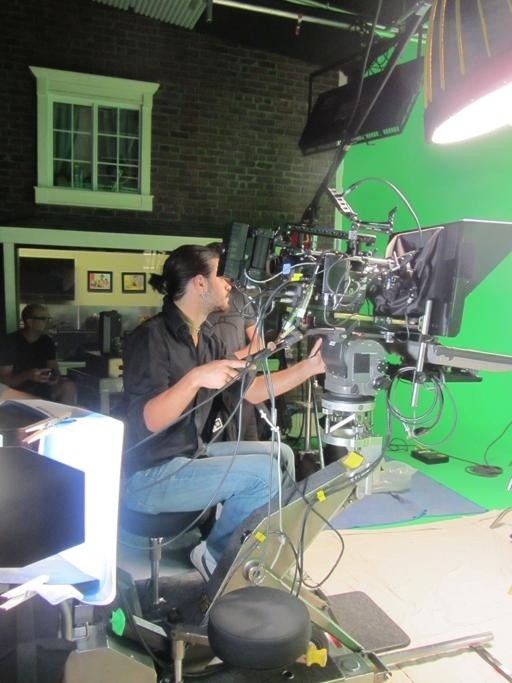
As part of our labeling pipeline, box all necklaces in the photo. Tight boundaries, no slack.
[185,317,202,333]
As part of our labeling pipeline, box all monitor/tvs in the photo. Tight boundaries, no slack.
[387,217,512,338]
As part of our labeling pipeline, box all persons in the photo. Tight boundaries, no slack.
[0,300,80,409]
[117,242,329,586]
[192,241,268,442]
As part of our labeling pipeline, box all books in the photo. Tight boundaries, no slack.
[0,399,74,447]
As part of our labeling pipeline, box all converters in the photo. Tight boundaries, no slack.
[411,448,449,464]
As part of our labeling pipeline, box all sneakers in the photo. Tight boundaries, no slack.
[190,541,219,583]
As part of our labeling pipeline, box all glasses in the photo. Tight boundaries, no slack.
[32,316,54,323]
